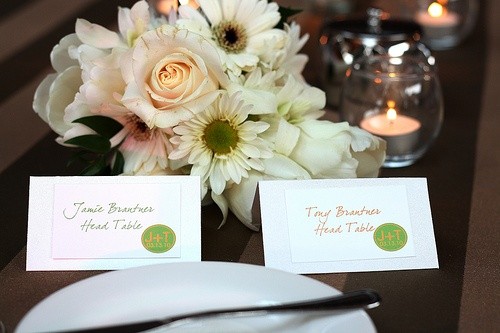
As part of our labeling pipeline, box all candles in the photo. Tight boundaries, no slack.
[414,3,458,39]
[360,108,421,154]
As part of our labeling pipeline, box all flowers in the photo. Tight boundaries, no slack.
[32,0,386,232]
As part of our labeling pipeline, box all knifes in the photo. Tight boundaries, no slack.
[46,288,383,333]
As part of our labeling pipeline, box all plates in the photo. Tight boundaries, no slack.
[14,261,379,333]
